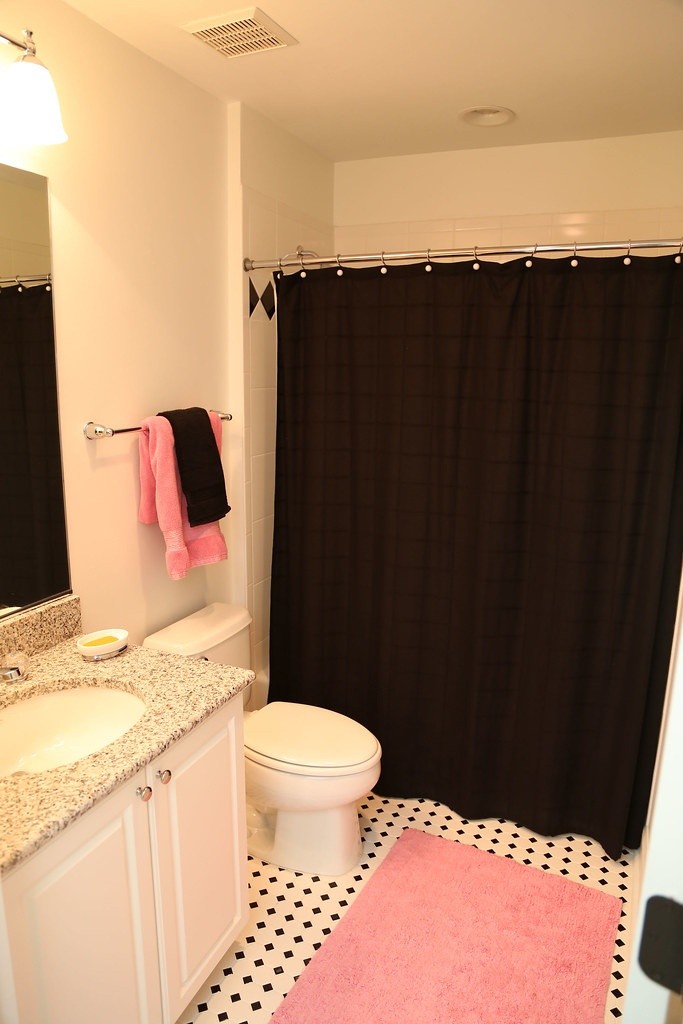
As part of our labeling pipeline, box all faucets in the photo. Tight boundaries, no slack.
[0,664,31,686]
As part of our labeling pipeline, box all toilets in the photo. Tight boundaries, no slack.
[140,600,383,876]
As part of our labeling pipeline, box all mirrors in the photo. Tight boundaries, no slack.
[0,163,73,622]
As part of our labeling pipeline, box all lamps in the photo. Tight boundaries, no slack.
[4,29,68,154]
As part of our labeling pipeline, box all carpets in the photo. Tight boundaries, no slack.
[267,827,622,1024]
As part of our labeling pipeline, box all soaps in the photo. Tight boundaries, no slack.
[84,634,117,647]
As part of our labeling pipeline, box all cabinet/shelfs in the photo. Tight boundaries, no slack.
[0,682,256,1024]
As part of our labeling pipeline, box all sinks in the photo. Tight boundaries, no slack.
[0,687,146,778]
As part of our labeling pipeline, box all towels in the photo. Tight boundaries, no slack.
[156,407,230,528]
[139,409,228,582]
